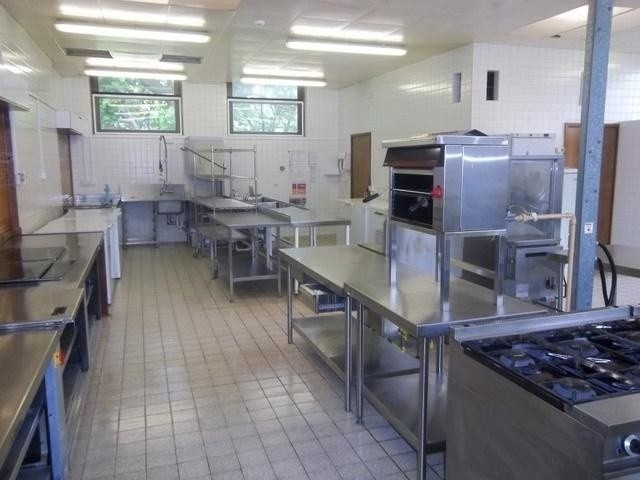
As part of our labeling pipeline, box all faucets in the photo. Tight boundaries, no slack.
[159,135,168,186]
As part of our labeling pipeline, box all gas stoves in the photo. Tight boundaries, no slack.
[461,315,640,415]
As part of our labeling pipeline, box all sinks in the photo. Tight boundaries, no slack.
[157,195,183,214]
